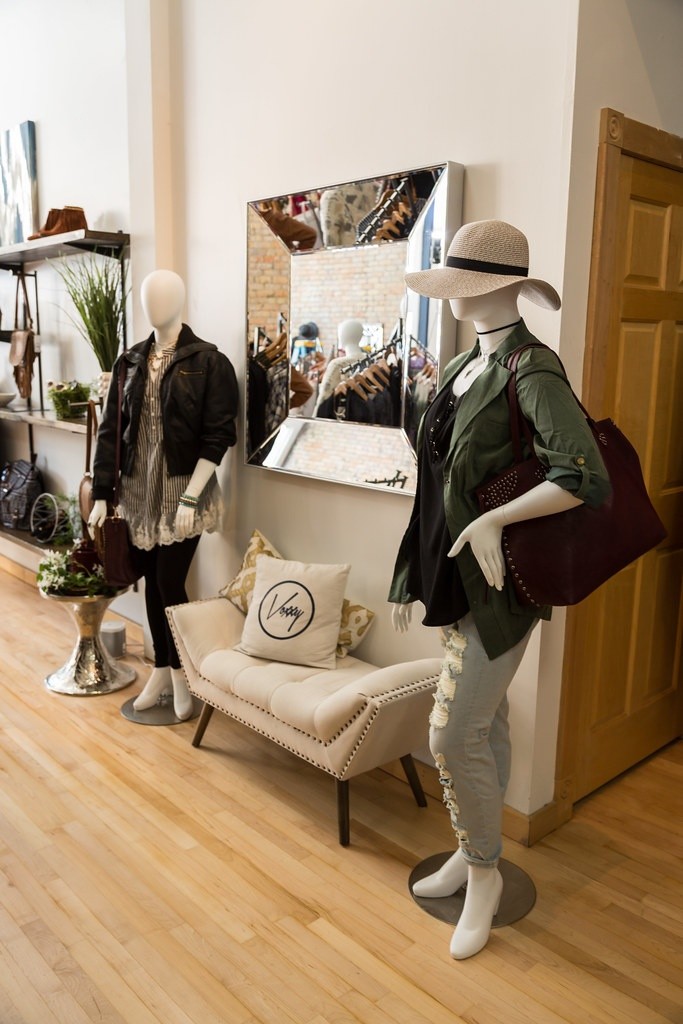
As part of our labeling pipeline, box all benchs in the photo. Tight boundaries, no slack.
[165,596,447,847]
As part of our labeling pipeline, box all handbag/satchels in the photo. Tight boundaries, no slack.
[472,342,670,610]
[9,330,35,398]
[100,517,133,588]
[0,452,44,530]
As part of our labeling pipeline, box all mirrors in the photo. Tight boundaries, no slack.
[243,161,465,496]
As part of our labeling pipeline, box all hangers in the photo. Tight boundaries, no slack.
[363,354,389,386]
[357,180,418,243]
[346,363,367,400]
[407,340,438,386]
[255,326,289,370]
[335,367,347,395]
[386,340,397,366]
[296,351,327,383]
[376,348,390,374]
[351,358,384,390]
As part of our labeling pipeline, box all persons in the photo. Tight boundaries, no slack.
[84,268,239,721]
[291,366,314,407]
[388,217,611,959]
[313,319,367,417]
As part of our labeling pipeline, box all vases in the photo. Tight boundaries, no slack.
[52,391,91,420]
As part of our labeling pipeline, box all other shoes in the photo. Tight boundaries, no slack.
[27,207,89,240]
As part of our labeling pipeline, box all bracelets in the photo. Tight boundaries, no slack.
[179,494,199,509]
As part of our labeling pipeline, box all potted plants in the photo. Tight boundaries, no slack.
[45,243,132,402]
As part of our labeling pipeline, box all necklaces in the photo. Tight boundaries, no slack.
[465,318,522,379]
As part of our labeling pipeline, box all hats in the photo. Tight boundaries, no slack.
[298,322,319,342]
[406,218,562,311]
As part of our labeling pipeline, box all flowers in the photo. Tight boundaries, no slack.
[47,378,93,399]
[35,538,99,598]
[0,228,130,573]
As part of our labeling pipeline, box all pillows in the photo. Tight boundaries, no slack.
[218,527,375,660]
[232,553,351,670]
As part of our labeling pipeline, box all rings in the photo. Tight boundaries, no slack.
[87,522,91,527]
